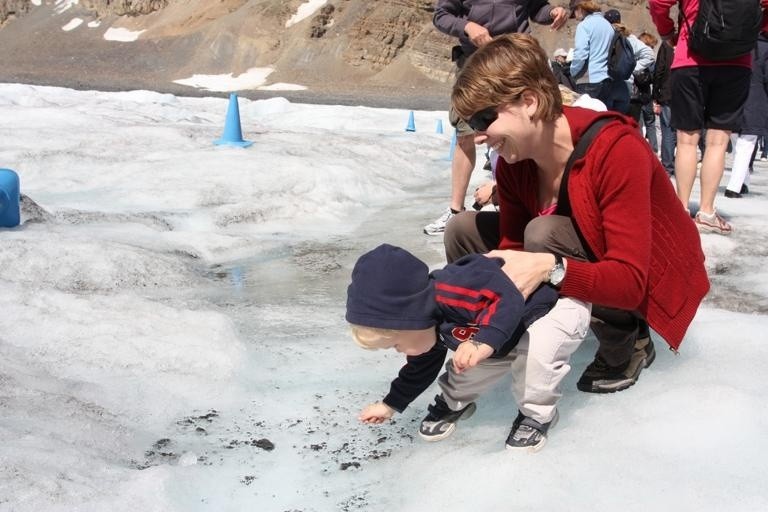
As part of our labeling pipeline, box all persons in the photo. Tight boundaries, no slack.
[421,1,568,238]
[443,34,711,394]
[550,0,767,235]
[344,242,592,453]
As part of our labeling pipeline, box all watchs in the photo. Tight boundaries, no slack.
[546,252,565,287]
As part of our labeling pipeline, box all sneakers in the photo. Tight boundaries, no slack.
[418,393,477,441]
[506,409,559,452]
[424,208,463,236]
[695,208,731,234]
[577,341,655,392]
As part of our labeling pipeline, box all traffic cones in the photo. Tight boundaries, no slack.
[435,119,443,134]
[211,90,253,148]
[405,111,418,133]
[443,129,457,162]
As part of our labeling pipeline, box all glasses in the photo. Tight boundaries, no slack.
[459,104,499,131]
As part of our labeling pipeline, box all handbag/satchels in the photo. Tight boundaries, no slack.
[607,27,637,80]
[633,68,652,86]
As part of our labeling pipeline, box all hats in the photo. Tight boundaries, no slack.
[553,47,568,57]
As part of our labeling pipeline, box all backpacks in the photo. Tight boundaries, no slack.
[688,0,764,61]
